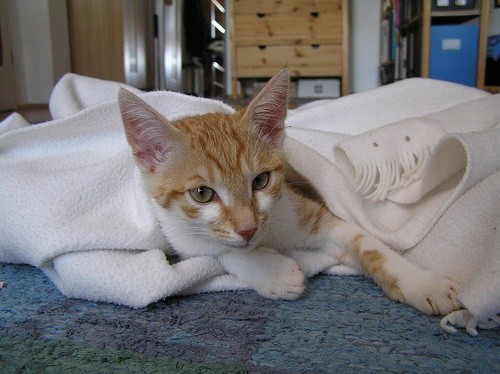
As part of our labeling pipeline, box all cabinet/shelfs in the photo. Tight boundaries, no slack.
[68,0,352,111]
[380,1,500,98]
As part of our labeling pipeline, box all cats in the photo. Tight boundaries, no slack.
[117,67,464,318]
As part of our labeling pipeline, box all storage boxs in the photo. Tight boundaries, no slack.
[298,79,341,99]
